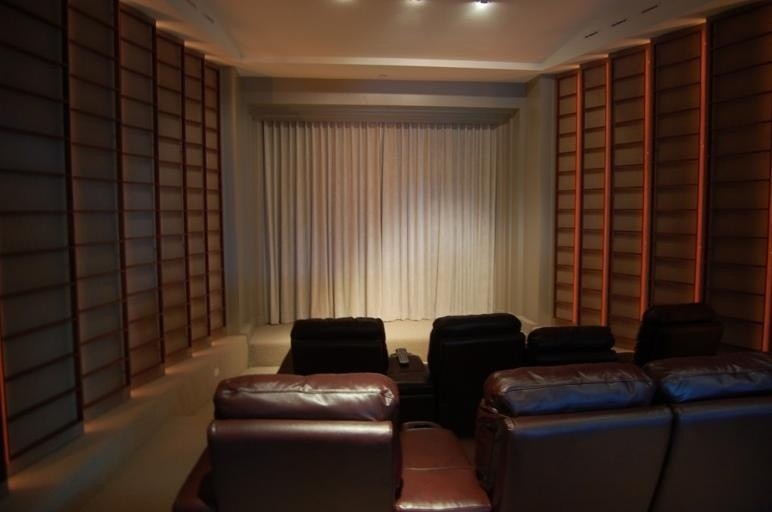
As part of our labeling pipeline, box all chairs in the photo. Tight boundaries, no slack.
[631,302,725,361]
[389,354,429,421]
[170,373,491,508]
[429,311,524,426]
[528,325,616,364]
[474,361,674,509]
[273,316,386,374]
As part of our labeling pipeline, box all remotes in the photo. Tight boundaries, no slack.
[396,348,409,364]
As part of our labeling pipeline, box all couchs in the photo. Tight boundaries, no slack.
[644,349,768,511]
